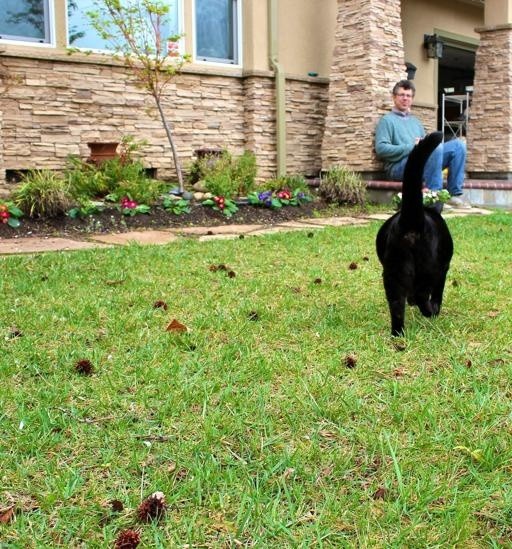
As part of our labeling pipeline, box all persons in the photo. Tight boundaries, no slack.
[375,79,473,209]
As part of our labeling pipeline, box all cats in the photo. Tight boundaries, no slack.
[376,131,453,341]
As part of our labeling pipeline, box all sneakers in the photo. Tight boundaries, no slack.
[448,196,472,209]
[443,202,453,210]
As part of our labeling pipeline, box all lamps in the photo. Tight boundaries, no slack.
[424,33,445,58]
